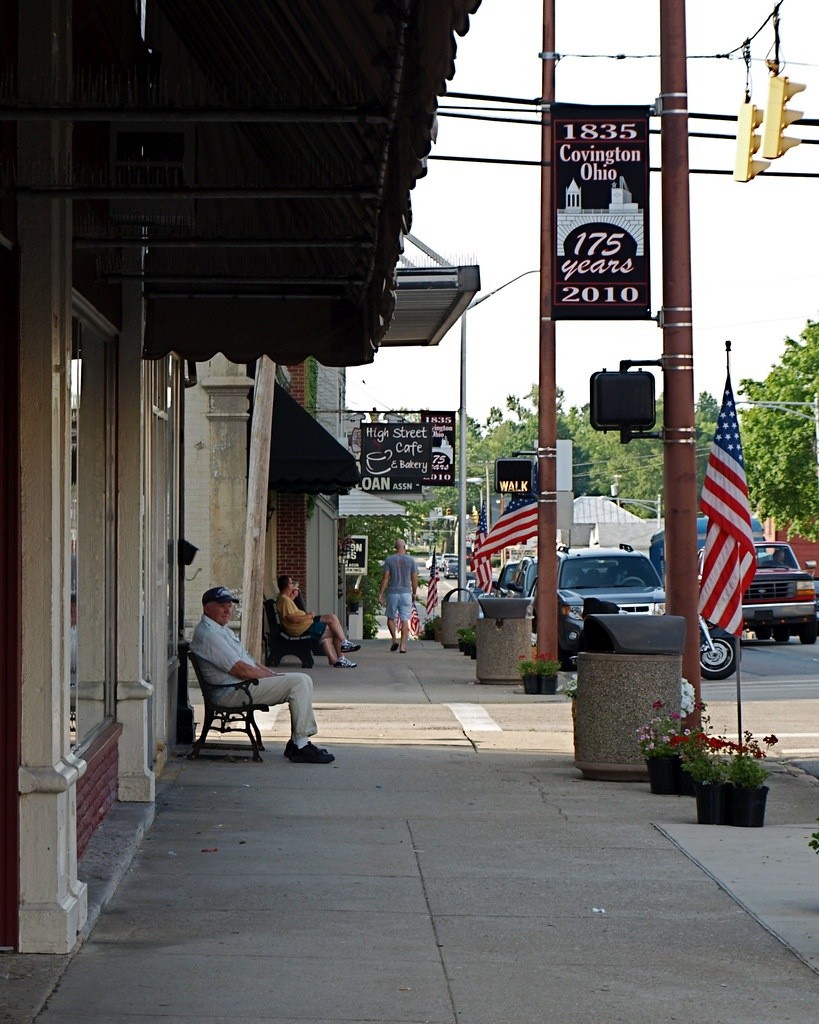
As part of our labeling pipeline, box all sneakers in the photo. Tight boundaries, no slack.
[284,738,335,764]
[335,657,356,668]
[341,639,361,653]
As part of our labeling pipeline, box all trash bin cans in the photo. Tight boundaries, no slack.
[440,588,479,649]
[573,614,686,786]
[476,598,534,684]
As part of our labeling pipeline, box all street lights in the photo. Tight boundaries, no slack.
[598,494,661,532]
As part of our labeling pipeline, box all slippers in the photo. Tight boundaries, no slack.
[400,646,407,654]
[391,643,398,651]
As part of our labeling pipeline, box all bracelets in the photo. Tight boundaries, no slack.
[413,594,417,596]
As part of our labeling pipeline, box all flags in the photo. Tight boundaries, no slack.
[473,508,493,592]
[698,377,757,636]
[427,549,440,614]
[397,604,423,636]
[470,463,538,572]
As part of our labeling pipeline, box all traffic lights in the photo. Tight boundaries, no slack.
[762,77,807,160]
[734,104,771,183]
[493,458,532,494]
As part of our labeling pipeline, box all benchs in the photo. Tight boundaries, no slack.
[263,598,318,668]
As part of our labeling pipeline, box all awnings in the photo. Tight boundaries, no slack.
[147,1,480,370]
[275,381,361,496]
[339,489,406,516]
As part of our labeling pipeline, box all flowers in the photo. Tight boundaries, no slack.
[635,677,778,789]
[345,587,362,603]
[455,621,477,644]
[554,673,579,698]
[517,653,562,676]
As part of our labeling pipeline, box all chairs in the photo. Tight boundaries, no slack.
[186,651,270,762]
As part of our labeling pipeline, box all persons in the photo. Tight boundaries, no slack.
[190,587,335,763]
[773,551,784,565]
[379,540,419,653]
[277,575,361,667]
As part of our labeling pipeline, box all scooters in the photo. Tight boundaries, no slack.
[695,611,742,681]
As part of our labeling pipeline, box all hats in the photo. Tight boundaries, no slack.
[202,585,239,604]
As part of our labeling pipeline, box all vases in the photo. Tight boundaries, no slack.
[694,784,769,828]
[671,754,696,797]
[348,601,359,613]
[521,673,557,695]
[458,639,476,659]
[644,755,672,795]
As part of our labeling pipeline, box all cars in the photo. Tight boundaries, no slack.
[465,571,496,619]
[425,552,460,579]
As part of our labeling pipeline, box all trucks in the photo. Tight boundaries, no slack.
[647,517,816,645]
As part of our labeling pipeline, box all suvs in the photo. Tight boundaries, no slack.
[554,544,667,669]
[490,552,538,629]
